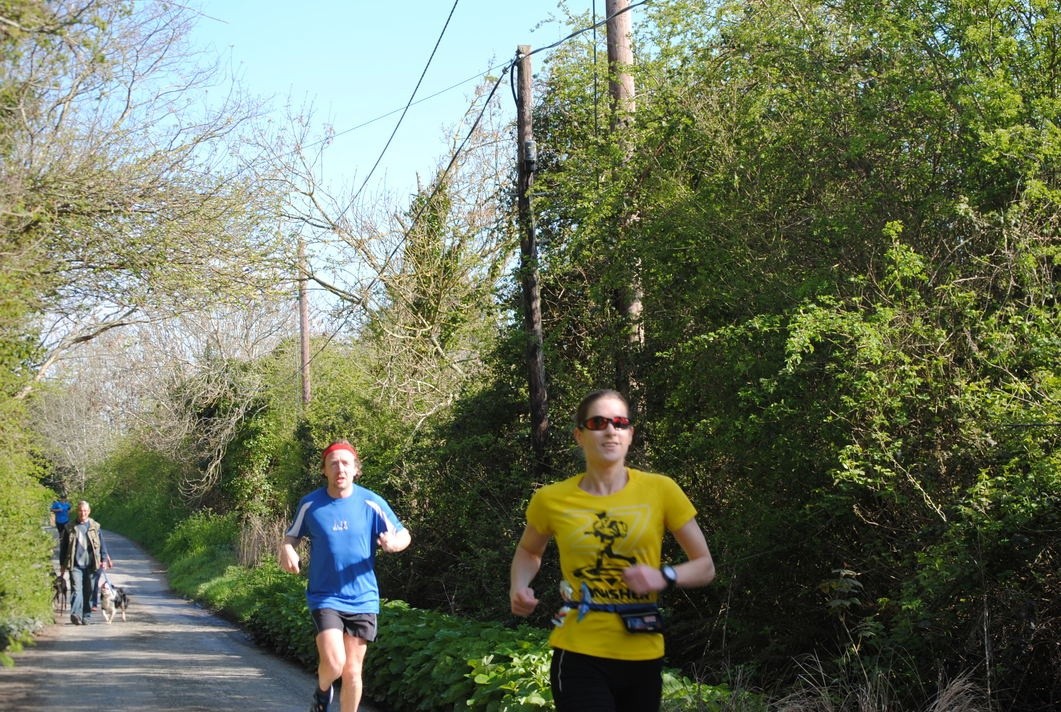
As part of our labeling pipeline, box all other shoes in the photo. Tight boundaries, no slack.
[91,608,97,611]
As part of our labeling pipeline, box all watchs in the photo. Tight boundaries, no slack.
[660,564,678,593]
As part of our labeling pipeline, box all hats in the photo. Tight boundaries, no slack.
[59,494,67,499]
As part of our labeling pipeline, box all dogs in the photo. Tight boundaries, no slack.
[55,576,68,617]
[101,581,130,624]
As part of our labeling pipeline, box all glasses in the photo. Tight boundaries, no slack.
[580,415,632,430]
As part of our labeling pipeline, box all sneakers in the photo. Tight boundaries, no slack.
[310,681,335,712]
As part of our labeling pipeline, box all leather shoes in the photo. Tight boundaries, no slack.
[82,617,90,624]
[71,614,82,624]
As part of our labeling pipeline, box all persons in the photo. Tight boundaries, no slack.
[58,500,103,626]
[508,388,718,712]
[90,526,108,612]
[49,492,72,539]
[280,441,413,712]
